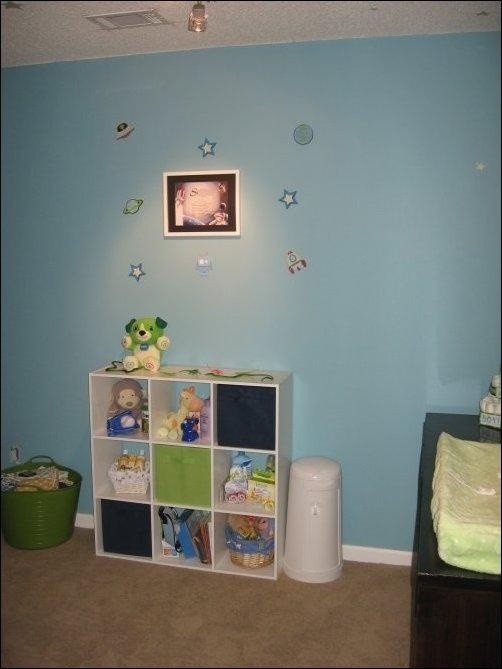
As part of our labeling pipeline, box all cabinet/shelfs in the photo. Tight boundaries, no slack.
[89,362,293,581]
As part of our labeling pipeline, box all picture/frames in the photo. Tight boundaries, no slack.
[163,170,241,237]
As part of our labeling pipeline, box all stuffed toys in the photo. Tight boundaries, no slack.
[121,317,170,372]
[228,514,274,542]
[157,386,204,441]
[179,416,199,441]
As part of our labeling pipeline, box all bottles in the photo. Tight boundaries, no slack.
[265,454,275,473]
[135,450,145,467]
[118,450,129,471]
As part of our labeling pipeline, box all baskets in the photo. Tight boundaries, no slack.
[224,526,274,569]
[107,461,150,494]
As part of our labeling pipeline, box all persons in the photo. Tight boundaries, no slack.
[174,182,185,226]
[105,378,143,436]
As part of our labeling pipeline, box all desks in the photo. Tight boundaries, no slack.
[410,413,502,668]
